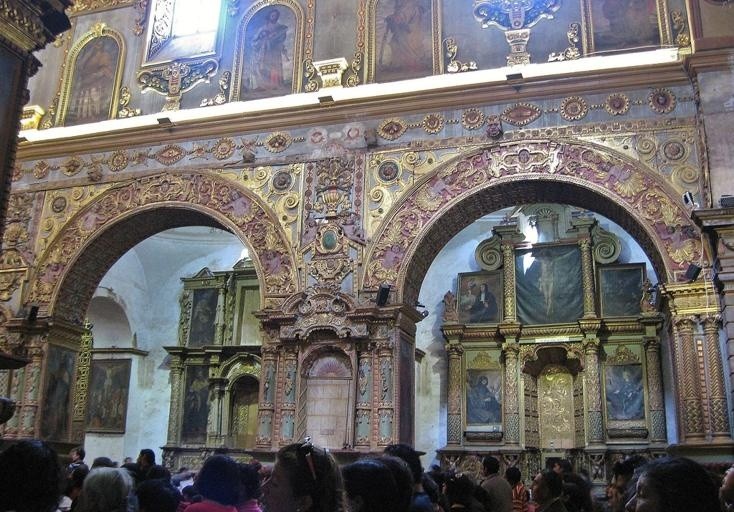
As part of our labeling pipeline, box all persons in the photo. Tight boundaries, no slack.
[472,376,501,424]
[612,367,644,420]
[250,8,289,91]
[194,299,214,346]
[519,248,580,317]
[379,0,431,72]
[89,362,127,431]
[77,39,112,120]
[639,278,657,312]
[188,370,209,435]
[464,283,498,323]
[1,436,733,512]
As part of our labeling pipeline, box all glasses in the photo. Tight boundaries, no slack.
[297,437,318,482]
